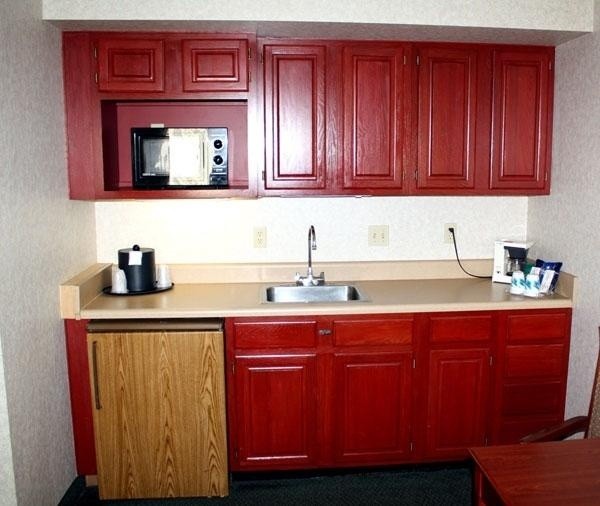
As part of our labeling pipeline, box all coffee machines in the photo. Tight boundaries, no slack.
[492,240,536,284]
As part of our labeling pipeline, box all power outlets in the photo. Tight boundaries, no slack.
[368,225,388,247]
[444,224,457,244]
[253,227,267,249]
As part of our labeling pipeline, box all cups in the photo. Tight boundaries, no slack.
[524,275,541,297]
[111,270,127,294]
[157,265,171,287]
[510,270,525,295]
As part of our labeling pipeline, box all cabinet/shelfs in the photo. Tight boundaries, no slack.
[485,43,556,195]
[225,316,328,472]
[328,310,422,469]
[411,43,484,194]
[421,309,500,464]
[496,307,571,444]
[334,39,411,197]
[61,31,260,201]
[260,38,334,198]
[62,318,231,501]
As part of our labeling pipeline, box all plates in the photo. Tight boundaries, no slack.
[101,281,175,295]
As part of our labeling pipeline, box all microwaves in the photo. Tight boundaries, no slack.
[132,126,229,189]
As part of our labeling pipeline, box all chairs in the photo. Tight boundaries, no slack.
[470,324,600,506]
